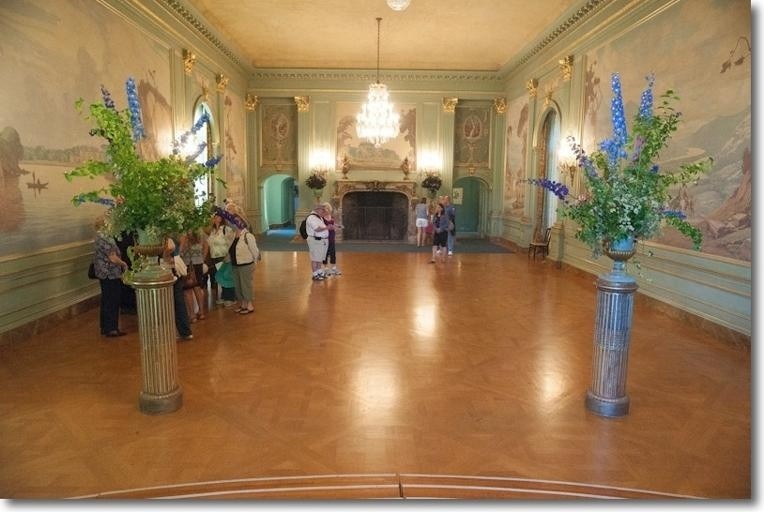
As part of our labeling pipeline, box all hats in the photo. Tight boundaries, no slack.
[216,264,237,288]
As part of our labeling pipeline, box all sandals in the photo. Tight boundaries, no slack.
[312,272,327,280]
[189,312,206,323]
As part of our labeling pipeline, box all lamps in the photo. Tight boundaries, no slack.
[387,0,411,11]
[355,18,402,149]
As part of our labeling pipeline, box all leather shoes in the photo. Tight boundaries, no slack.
[101,327,128,338]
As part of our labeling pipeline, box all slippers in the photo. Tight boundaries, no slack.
[235,307,255,314]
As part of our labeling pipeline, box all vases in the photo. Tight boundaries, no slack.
[135,228,164,246]
[610,234,637,252]
[312,189,323,197]
[427,188,438,199]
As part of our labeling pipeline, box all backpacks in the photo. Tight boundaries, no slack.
[299,213,323,240]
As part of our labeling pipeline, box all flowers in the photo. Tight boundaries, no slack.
[526,70,713,282]
[304,171,327,189]
[63,78,228,284]
[421,171,442,192]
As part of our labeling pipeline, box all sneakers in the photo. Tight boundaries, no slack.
[325,267,342,276]
[437,249,442,254]
[175,332,194,340]
[448,251,453,255]
[217,298,238,307]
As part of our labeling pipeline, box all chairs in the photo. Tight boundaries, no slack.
[528,228,551,260]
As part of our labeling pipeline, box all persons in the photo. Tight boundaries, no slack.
[88,202,259,344]
[306,203,337,282]
[414,197,429,250]
[444,196,455,256]
[317,202,344,277]
[426,202,450,266]
[434,195,445,254]
